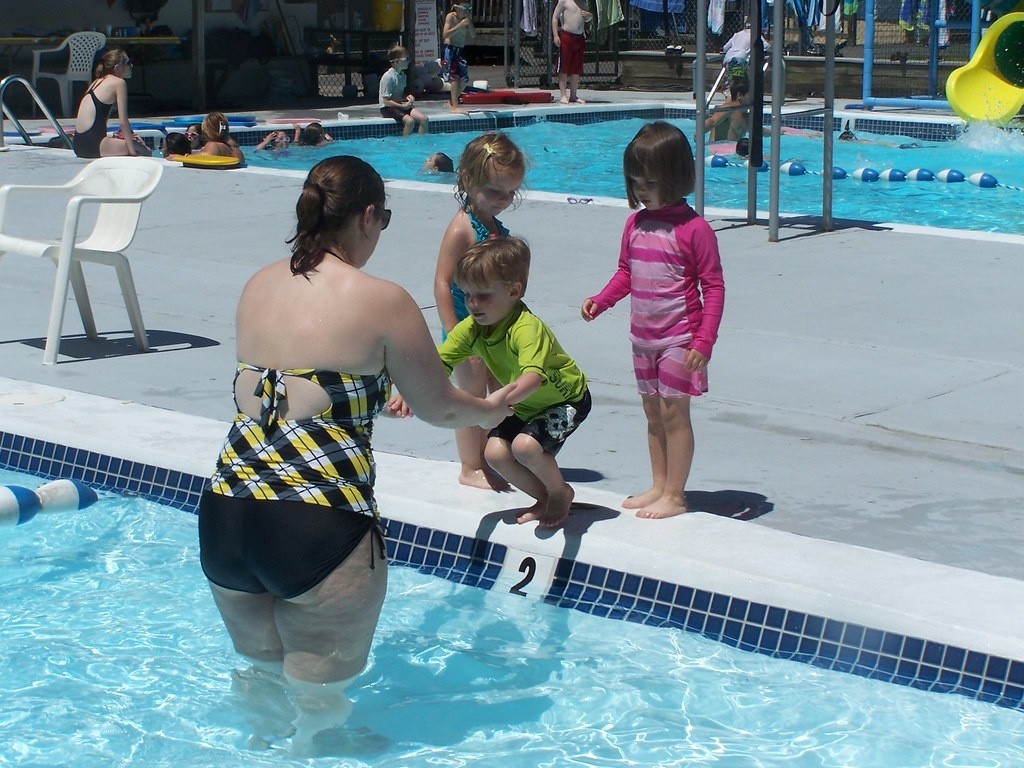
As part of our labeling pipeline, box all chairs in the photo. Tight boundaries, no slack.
[30,31,108,117]
[0,155,164,365]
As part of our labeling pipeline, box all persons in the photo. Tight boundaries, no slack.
[693,80,771,143]
[379,42,429,136]
[424,152,454,173]
[723,15,771,91]
[257,130,289,149]
[198,155,518,683]
[552,0,594,104]
[161,111,245,164]
[73,44,152,159]
[384,233,591,527]
[582,120,725,520]
[736,138,748,160]
[840,132,937,148]
[291,120,333,146]
[442,0,477,115]
[434,132,532,489]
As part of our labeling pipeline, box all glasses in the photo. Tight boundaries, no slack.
[185,130,201,139]
[454,4,473,11]
[389,55,410,62]
[113,58,131,71]
[353,204,392,230]
[272,135,291,143]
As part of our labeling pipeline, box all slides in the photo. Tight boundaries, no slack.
[945,11,1024,125]
